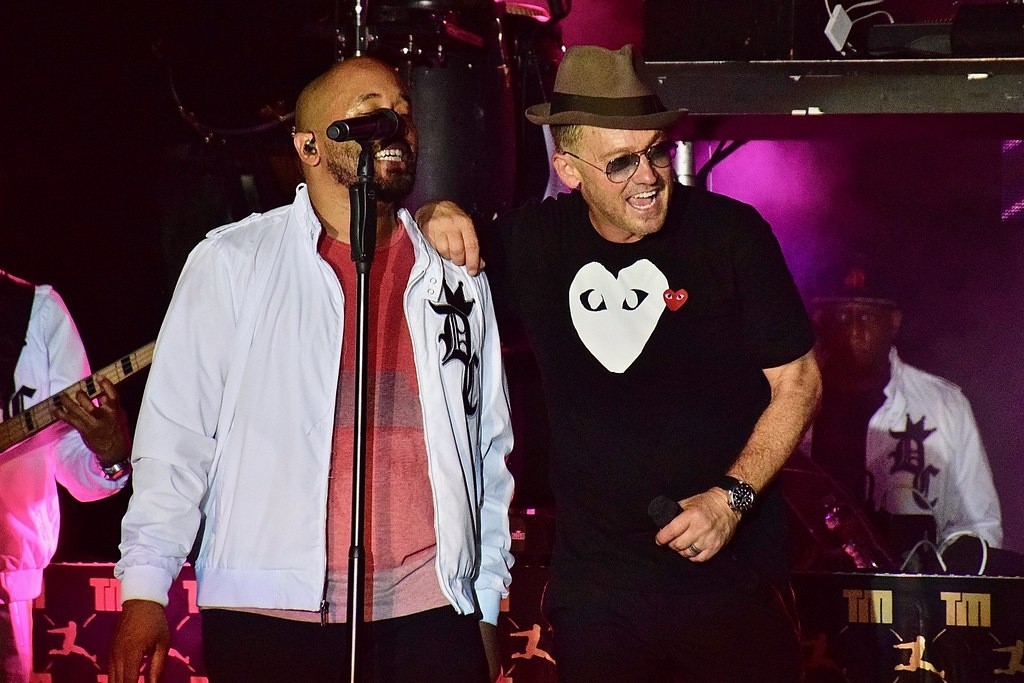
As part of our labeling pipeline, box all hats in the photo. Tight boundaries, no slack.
[524,43,678,130]
[810,270,894,305]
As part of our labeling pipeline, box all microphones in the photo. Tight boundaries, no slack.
[326,107,408,146]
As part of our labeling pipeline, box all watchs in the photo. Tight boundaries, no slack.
[714,475,757,513]
[101,460,131,481]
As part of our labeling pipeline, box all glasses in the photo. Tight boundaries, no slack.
[562,140,678,184]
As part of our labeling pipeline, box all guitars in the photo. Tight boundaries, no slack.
[0,340,156,452]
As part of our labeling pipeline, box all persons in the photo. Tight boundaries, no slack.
[0,269,132,683]
[107,58,515,683]
[809,261,1004,549]
[415,46,823,683]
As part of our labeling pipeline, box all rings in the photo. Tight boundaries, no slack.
[688,545,701,555]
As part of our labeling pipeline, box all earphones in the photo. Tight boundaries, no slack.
[303,131,318,155]
